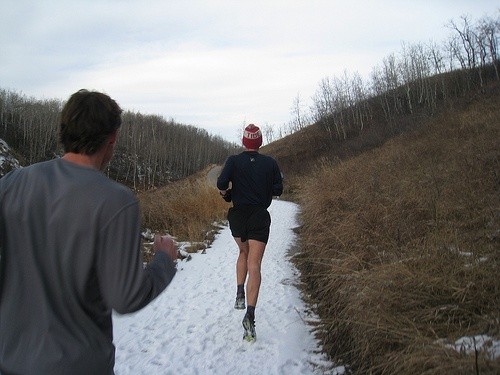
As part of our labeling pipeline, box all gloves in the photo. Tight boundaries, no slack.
[220,191,231,202]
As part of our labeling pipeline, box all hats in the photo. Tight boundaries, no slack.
[242,123,262,149]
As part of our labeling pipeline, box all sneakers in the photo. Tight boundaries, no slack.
[243,313,256,342]
[234,295,246,310]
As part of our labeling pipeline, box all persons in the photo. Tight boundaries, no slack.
[0,88,177,375]
[216,124,283,343]
